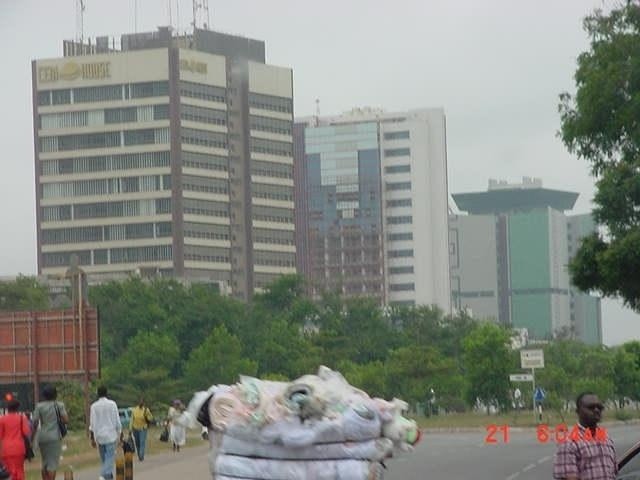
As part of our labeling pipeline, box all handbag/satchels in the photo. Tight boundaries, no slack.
[160,429,169,442]
[22,436,34,459]
[127,432,136,452]
[59,422,67,437]
[143,408,156,424]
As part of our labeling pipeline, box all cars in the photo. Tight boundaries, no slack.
[616,441,640,480]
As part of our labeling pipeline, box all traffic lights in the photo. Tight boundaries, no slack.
[4,392,14,401]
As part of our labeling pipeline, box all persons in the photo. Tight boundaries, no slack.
[89,386,124,480]
[553,392,618,480]
[0,386,69,480]
[167,399,187,452]
[129,395,152,462]
